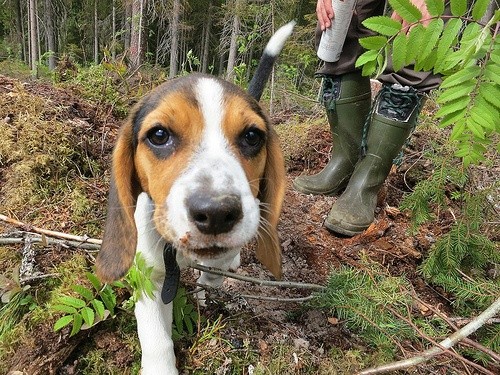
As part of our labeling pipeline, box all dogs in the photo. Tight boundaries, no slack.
[94,19,297,375]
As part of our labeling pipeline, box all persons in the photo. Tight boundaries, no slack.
[292,0,478,236]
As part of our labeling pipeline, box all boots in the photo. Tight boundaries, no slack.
[292,71,429,237]
